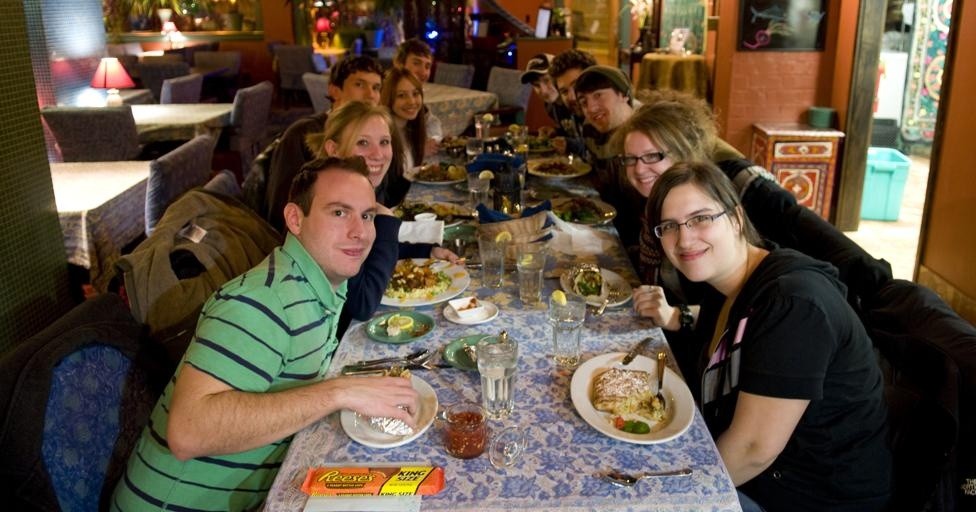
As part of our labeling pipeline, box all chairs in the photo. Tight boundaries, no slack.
[3,292,160,509]
[433,62,475,88]
[145,134,217,236]
[39,36,362,184]
[486,67,532,126]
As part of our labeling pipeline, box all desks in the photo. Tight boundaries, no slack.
[424,82,500,139]
[269,137,743,511]
[50,161,150,296]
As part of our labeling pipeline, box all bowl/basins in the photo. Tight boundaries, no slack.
[446,146,464,158]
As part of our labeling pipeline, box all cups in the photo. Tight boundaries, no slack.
[467,172,489,206]
[494,168,523,217]
[443,400,486,458]
[503,240,527,289]
[513,146,528,171]
[516,243,546,305]
[477,335,518,421]
[474,115,492,139]
[511,126,528,144]
[549,294,586,367]
[414,212,437,221]
[478,232,503,289]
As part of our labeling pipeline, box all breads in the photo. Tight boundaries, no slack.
[593,367,649,414]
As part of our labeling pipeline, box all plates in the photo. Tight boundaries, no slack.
[550,197,617,225]
[365,310,435,345]
[442,334,519,372]
[560,265,634,307]
[448,296,485,319]
[403,165,467,185]
[526,157,592,181]
[547,221,614,257]
[512,136,553,152]
[527,182,600,200]
[388,201,469,229]
[339,373,438,449]
[380,257,470,308]
[435,136,471,152]
[443,300,499,325]
[570,351,695,447]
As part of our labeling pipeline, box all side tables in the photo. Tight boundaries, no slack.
[749,123,845,221]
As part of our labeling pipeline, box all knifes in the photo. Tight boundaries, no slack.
[621,335,656,366]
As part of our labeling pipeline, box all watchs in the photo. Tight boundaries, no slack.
[675,303,695,335]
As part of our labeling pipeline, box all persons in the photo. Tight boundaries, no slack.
[301,100,459,321]
[521,48,898,511]
[392,38,444,154]
[374,67,423,178]
[264,54,398,231]
[106,152,422,511]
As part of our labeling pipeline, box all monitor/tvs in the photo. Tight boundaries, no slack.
[533,6,554,39]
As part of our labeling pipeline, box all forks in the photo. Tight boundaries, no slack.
[655,353,667,411]
[358,349,429,368]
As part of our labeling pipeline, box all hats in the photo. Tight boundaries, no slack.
[578,65,631,96]
[521,54,556,84]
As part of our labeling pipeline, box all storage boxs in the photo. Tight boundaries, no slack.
[859,149,912,221]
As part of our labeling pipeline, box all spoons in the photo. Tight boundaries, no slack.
[342,350,439,374]
[602,468,692,486]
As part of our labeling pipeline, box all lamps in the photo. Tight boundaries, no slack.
[90,57,135,110]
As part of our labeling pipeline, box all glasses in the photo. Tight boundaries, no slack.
[618,152,664,167]
[654,212,725,238]
